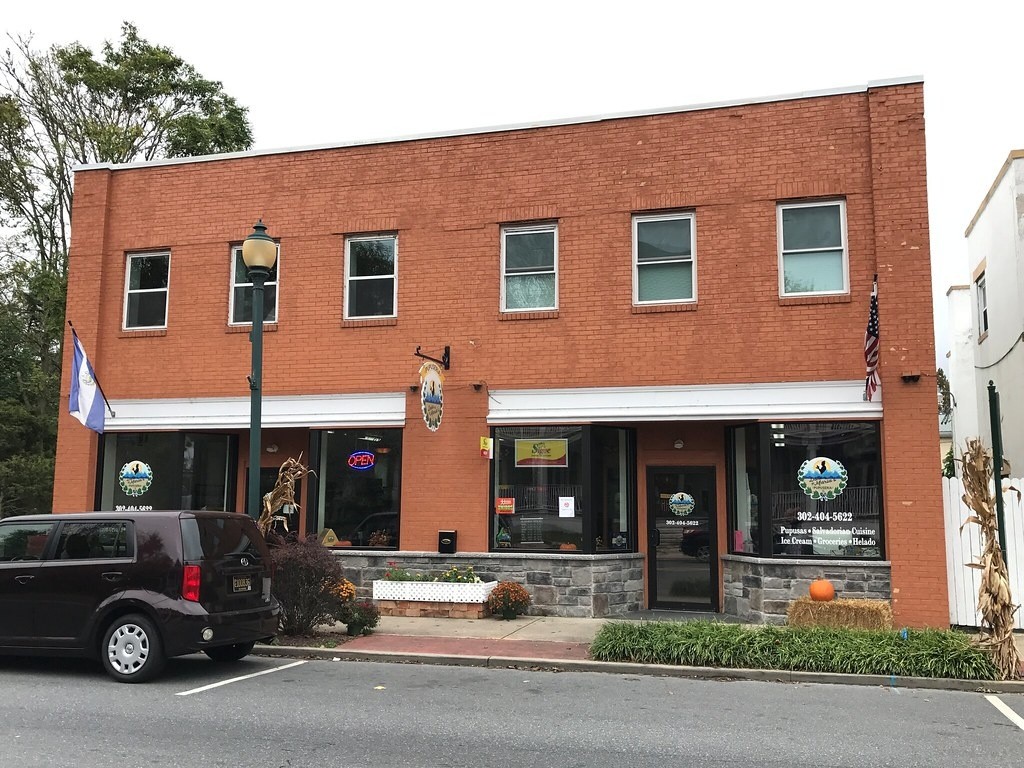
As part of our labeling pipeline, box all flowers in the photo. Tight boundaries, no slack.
[385,561,485,584]
[331,578,356,603]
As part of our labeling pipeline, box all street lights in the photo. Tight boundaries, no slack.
[242,216,277,523]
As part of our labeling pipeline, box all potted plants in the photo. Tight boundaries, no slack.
[488,581,531,620]
[368,529,392,546]
[338,598,381,636]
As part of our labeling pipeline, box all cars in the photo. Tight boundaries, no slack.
[679,519,782,564]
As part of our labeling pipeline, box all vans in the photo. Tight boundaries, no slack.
[0,509,282,685]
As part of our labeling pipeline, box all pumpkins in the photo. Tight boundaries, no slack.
[810,575,835,602]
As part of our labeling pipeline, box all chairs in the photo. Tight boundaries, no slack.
[65,533,90,558]
[3,531,29,560]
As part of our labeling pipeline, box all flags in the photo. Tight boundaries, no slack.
[862,273,881,402]
[69,330,105,434]
